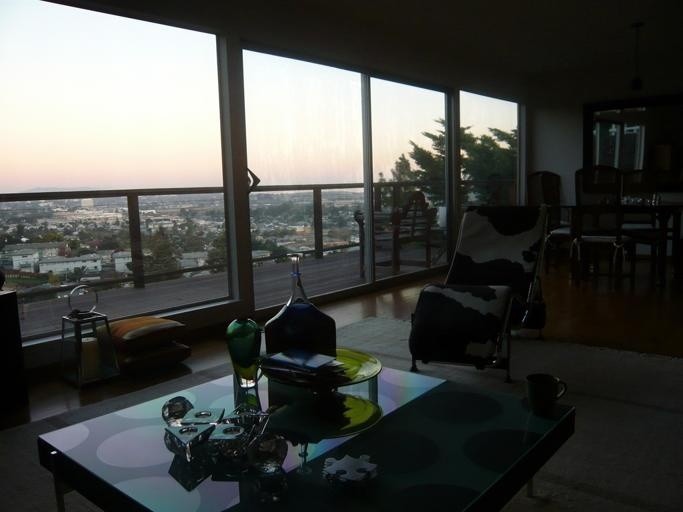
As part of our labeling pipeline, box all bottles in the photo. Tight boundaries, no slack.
[226,306,261,388]
[232,376,262,410]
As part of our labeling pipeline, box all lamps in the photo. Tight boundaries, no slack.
[629,22,644,91]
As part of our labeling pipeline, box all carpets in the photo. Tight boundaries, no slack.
[0,317,682,512]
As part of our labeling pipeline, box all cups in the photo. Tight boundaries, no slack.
[524,373,568,411]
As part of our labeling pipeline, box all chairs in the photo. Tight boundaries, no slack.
[527,171,560,264]
[409,204,548,384]
[619,168,667,288]
[574,165,619,284]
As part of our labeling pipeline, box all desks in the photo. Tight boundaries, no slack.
[549,201,683,292]
[37,352,577,512]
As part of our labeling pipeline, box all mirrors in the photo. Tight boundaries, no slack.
[582,93,683,193]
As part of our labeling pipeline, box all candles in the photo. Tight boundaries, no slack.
[80,336,100,371]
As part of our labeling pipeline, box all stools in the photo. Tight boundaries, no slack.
[97,316,191,377]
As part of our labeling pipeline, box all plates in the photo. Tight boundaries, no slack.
[264,392,384,439]
[261,347,382,389]
